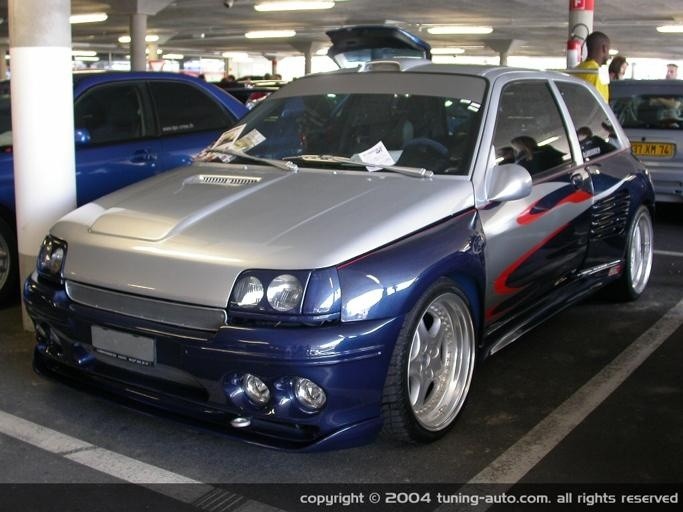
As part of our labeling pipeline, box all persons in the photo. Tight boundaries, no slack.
[228,75,235,82]
[608,55,628,81]
[666,64,678,80]
[264,73,271,80]
[274,74,282,80]
[511,136,566,174]
[570,31,611,104]
[578,127,616,160]
[496,147,516,166]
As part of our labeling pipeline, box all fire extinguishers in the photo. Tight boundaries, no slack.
[567,22,592,71]
